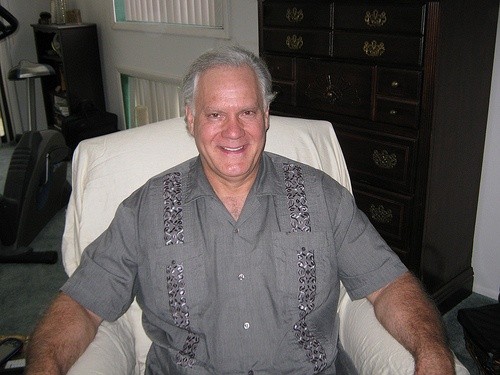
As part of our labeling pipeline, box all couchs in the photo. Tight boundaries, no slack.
[61,116,470,375]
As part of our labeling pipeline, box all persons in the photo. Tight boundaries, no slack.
[27,45,456,375]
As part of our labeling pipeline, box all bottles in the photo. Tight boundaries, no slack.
[56,0,65,25]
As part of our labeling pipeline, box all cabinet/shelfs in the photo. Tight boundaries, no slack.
[258,0,500,318]
[31,22,118,153]
